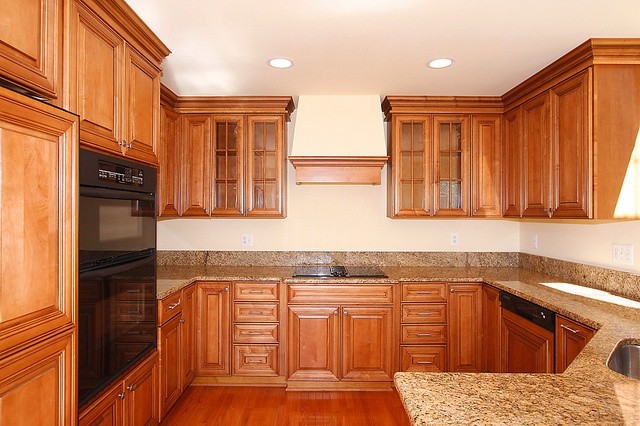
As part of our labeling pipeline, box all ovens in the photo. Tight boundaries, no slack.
[77,148,157,408]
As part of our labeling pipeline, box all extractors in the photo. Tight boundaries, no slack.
[289,97,390,185]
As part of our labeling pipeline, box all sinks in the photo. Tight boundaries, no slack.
[605,339,640,381]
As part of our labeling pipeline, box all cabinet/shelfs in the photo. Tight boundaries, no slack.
[287,283,397,391]
[1,88,77,426]
[78,276,106,388]
[520,40,640,222]
[472,97,504,221]
[130,201,155,218]
[484,283,554,372]
[557,314,597,373]
[0,0,61,108]
[179,97,213,218]
[159,82,179,220]
[107,278,155,375]
[503,83,520,218]
[157,280,196,421]
[284,153,390,185]
[381,96,472,218]
[212,97,294,219]
[190,280,288,387]
[62,1,172,168]
[77,351,159,426]
[401,283,483,372]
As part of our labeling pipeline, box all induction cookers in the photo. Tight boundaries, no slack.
[291,265,388,277]
